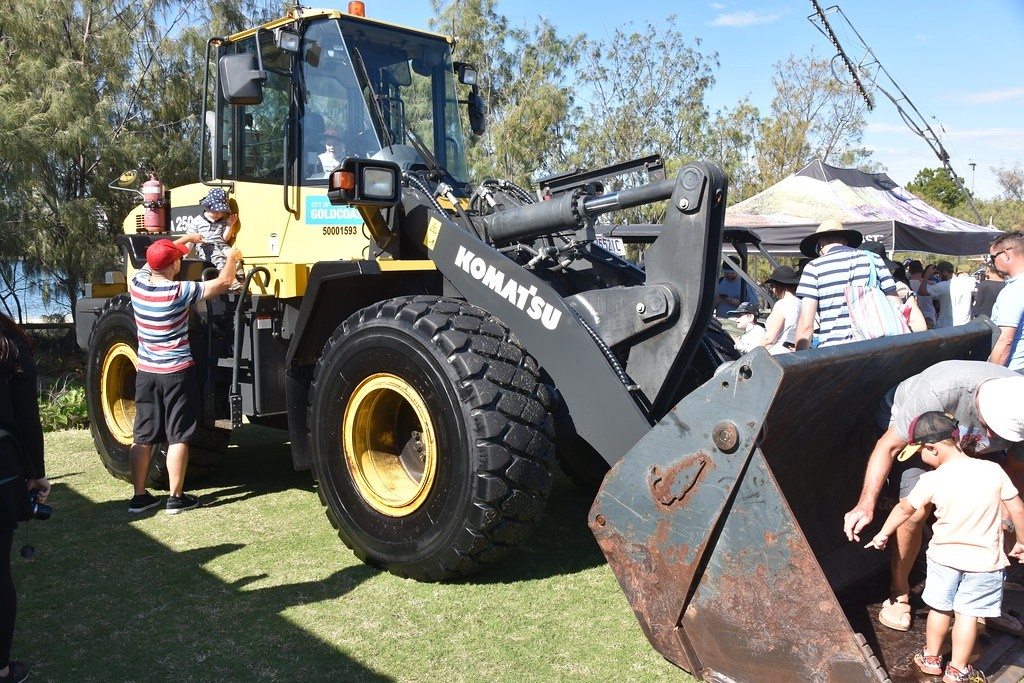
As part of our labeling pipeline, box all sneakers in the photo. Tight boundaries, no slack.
[943,662,988,683]
[0,662,30,683]
[128,491,162,513]
[165,493,200,514]
[915,646,943,675]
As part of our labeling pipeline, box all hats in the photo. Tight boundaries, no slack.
[979,376,1024,442]
[764,266,799,283]
[147,239,190,271]
[198,189,232,214]
[859,241,901,269]
[896,410,960,462]
[935,262,954,270]
[902,258,912,265]
[983,260,996,268]
[721,256,739,273]
[318,126,346,140]
[923,264,939,271]
[799,221,863,258]
[797,259,810,265]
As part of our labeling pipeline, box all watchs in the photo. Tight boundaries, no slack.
[1001,519,1015,534]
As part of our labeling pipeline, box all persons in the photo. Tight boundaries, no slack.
[863,410,1024,683]
[182,188,246,291]
[315,129,349,173]
[0,311,51,683]
[844,360,1024,631]
[713,221,1024,532]
[129,233,242,516]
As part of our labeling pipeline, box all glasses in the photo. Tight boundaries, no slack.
[990,247,1012,264]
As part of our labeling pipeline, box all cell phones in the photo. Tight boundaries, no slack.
[783,342,796,349]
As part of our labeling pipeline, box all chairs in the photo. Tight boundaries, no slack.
[266,112,325,180]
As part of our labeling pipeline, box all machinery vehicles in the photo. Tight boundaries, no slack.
[83,1,1024,683]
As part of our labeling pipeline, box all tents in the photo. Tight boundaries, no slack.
[722,159,1006,262]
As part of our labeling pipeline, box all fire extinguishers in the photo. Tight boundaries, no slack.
[142,172,167,234]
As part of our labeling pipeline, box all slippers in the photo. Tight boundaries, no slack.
[977,609,1024,634]
[879,598,911,631]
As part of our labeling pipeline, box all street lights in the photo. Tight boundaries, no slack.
[968,163,976,193]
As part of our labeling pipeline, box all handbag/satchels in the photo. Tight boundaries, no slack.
[843,251,912,342]
